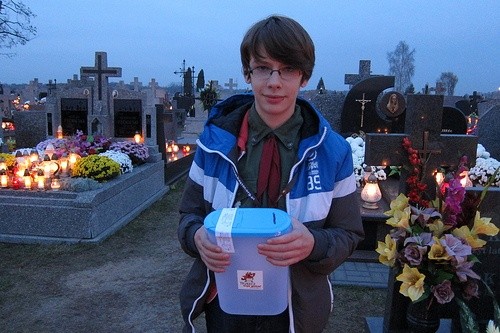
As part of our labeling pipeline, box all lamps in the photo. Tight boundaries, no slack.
[134,131,141,143]
[57,125,63,139]
[0,142,82,192]
[360,174,382,210]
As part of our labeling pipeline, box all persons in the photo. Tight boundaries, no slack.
[386,94,399,113]
[177,14,365,333]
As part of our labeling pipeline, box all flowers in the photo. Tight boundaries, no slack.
[468,143,500,187]
[0,133,151,183]
[376,136,500,332]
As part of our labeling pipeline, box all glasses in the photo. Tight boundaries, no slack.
[248,66,304,81]
